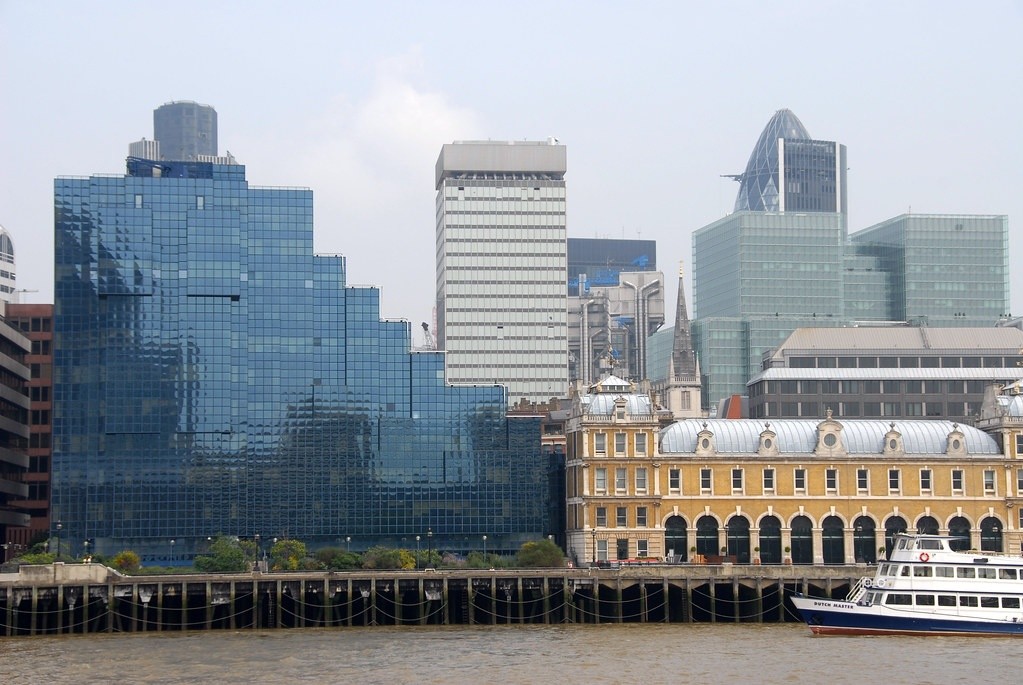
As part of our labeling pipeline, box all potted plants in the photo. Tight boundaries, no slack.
[784,546,790,565]
[690,548,697,562]
[754,547,759,564]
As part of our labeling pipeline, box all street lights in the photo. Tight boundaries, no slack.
[482,535,487,563]
[84,541,89,554]
[416,536,421,571]
[346,536,350,553]
[253,534,260,571]
[426,526,434,568]
[856,522,864,562]
[723,524,730,562]
[169,540,175,566]
[590,528,599,568]
[55,519,63,562]
[992,526,998,551]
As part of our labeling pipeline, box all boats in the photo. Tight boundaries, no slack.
[789,529,1023,639]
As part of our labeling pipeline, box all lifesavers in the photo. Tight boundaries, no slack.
[919,553,929,562]
[863,578,872,588]
[877,578,886,588]
